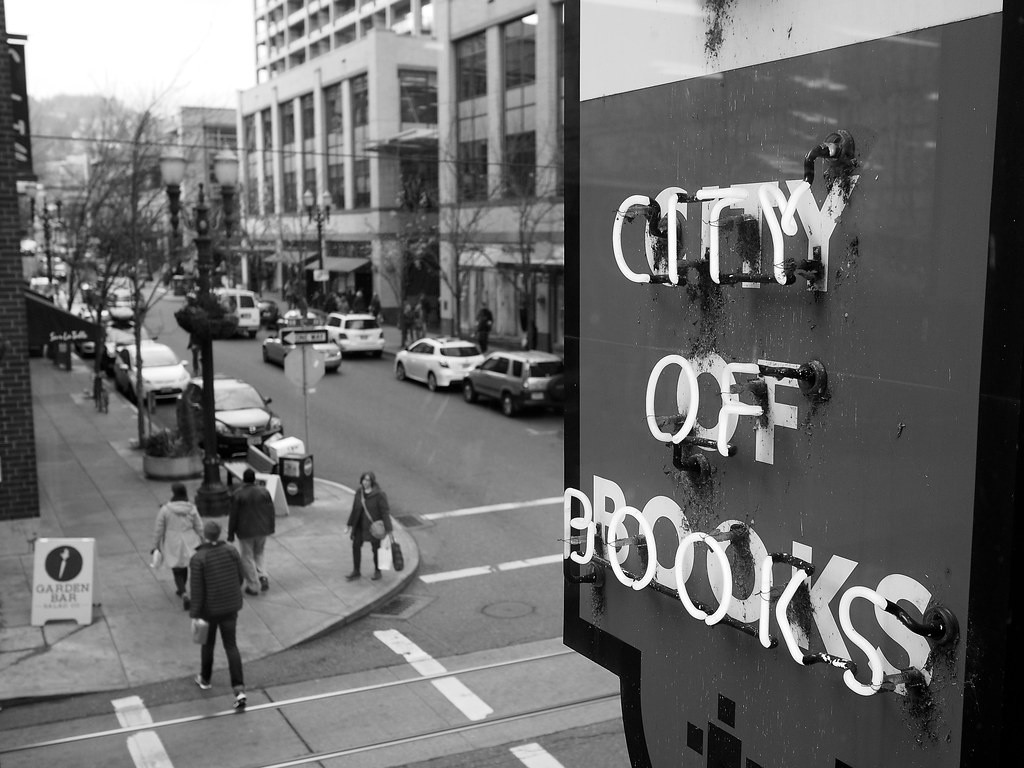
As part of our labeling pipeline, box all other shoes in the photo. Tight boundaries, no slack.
[184,598,190,610]
[345,570,361,579]
[245,585,258,596]
[372,569,380,580]
[260,576,268,591]
[175,589,180,596]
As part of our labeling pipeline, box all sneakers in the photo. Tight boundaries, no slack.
[195,675,211,690]
[233,693,246,709]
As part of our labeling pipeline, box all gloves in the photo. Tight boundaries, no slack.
[150,546,161,554]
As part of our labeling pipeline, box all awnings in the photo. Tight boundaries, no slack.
[23,290,108,346]
[262,251,316,263]
[304,255,370,273]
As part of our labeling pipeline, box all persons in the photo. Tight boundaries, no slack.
[150,482,208,611]
[256,274,264,285]
[282,272,382,328]
[189,520,247,709]
[516,298,538,350]
[344,471,393,581]
[470,301,494,354]
[398,293,427,348]
[227,468,275,595]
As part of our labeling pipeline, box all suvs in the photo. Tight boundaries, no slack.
[314,311,385,358]
[462,349,566,417]
[72,307,111,351]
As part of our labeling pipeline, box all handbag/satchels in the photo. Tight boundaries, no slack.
[369,520,386,540]
[388,531,403,570]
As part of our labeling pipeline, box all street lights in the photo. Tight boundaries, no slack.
[304,188,333,294]
[160,134,241,517]
[25,180,63,284]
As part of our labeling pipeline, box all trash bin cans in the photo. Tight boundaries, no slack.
[279,453,314,506]
[55,342,72,371]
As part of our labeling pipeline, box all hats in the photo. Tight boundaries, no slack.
[171,482,187,497]
[243,468,256,485]
[205,521,221,540]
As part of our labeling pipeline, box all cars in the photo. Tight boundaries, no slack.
[262,332,342,372]
[113,343,191,403]
[20,240,37,255]
[30,277,50,293]
[393,336,485,393]
[95,321,158,368]
[259,300,279,322]
[38,261,67,280]
[175,375,285,454]
[86,275,145,311]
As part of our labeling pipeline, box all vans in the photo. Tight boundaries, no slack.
[210,288,260,337]
